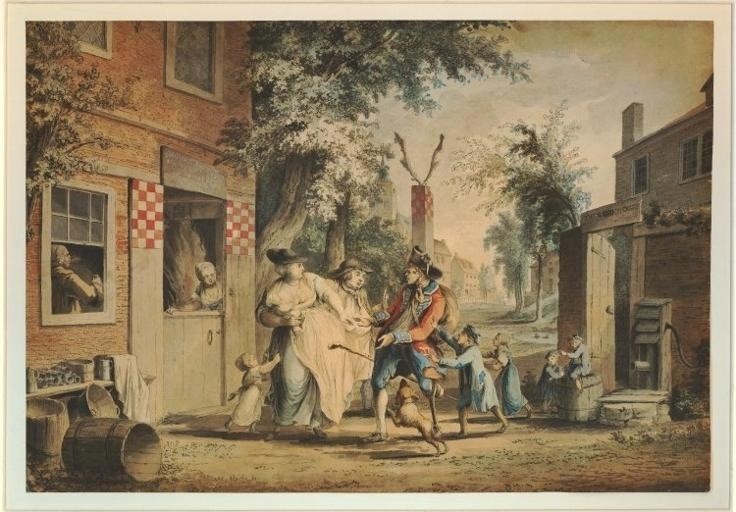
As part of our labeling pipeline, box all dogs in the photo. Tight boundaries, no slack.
[386,379,449,457]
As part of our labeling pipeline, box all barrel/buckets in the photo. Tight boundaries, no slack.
[26,397,69,466]
[555,371,603,422]
[59,416,164,484]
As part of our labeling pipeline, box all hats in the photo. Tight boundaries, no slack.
[328,258,374,279]
[403,246,443,278]
[267,248,308,264]
